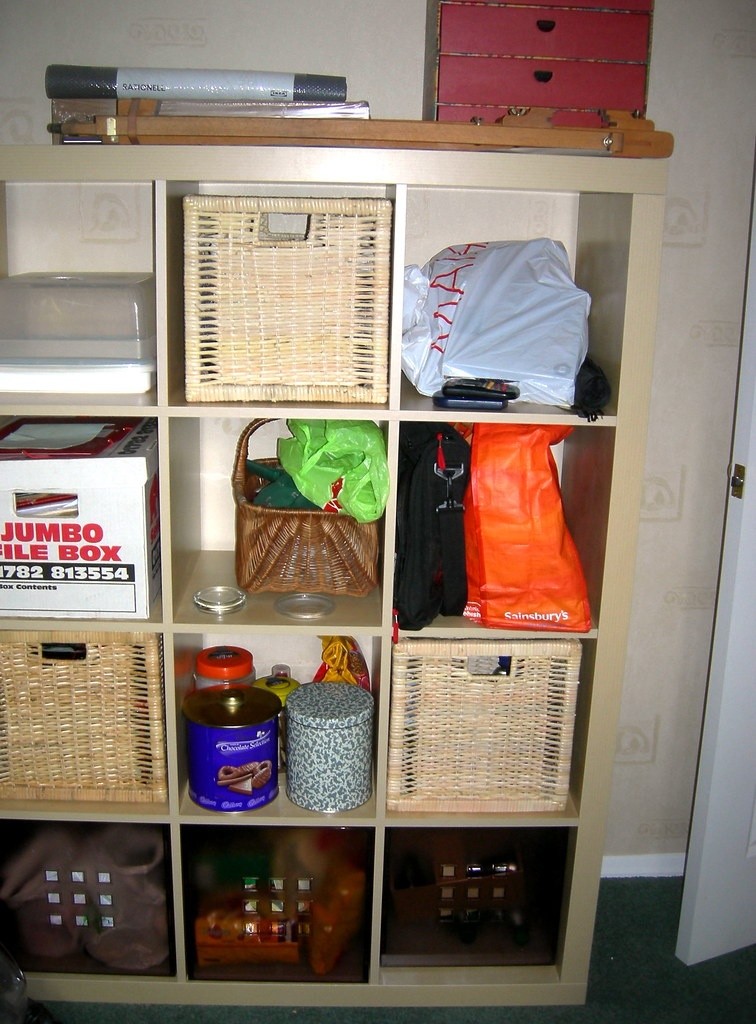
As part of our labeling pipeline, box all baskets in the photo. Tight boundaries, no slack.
[386,638,583,812]
[183,194,393,404]
[233,418,378,598]
[0,631,168,803]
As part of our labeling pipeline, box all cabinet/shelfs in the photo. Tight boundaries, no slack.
[428,0,655,129]
[0,145,665,1008]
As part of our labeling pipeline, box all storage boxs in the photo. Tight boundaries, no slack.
[0,417,162,619]
[0,272,157,395]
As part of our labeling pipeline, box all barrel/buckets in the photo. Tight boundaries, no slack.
[182,682,283,814]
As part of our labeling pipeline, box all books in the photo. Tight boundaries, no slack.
[0,415,149,519]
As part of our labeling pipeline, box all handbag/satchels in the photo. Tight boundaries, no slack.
[393,420,591,644]
[401,237,592,408]
